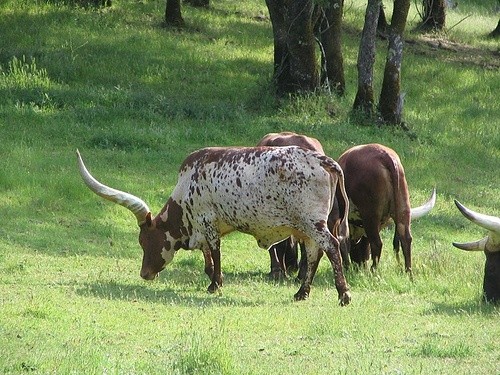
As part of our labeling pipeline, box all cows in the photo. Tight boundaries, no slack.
[74,146,352,307]
[254,131,437,287]
[452,199,500,305]
